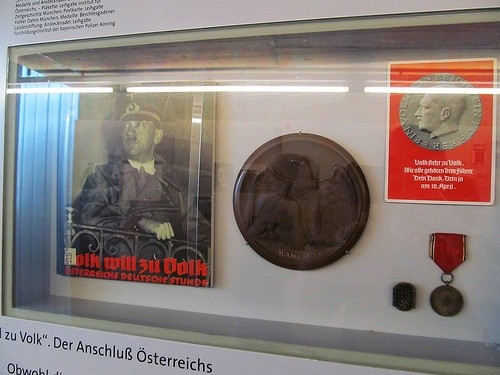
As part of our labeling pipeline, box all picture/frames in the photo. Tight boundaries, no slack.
[384,56,496,207]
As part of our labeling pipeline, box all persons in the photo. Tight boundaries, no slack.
[413,84,466,140]
[65,94,209,283]
